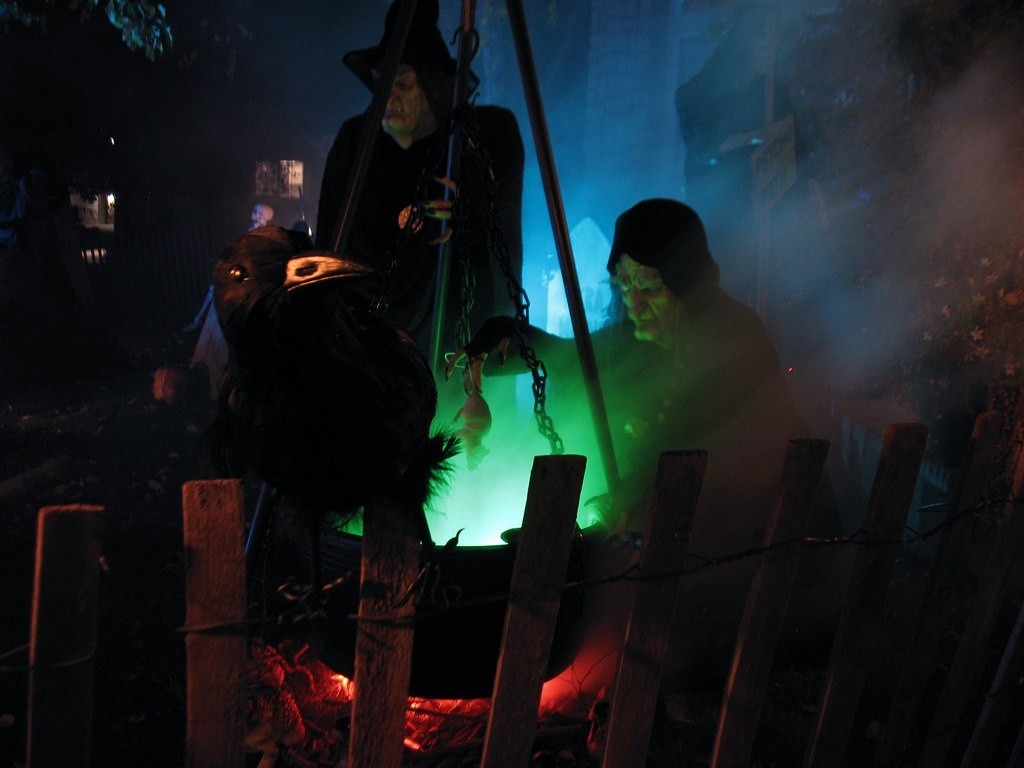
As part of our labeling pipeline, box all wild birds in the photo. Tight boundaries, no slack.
[187,226,461,647]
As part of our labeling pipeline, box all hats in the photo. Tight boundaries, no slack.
[607,198,720,298]
[340,0,480,98]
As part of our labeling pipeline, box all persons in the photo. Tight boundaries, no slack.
[442,197,791,536]
[314,4,523,371]
[676,0,834,416]
